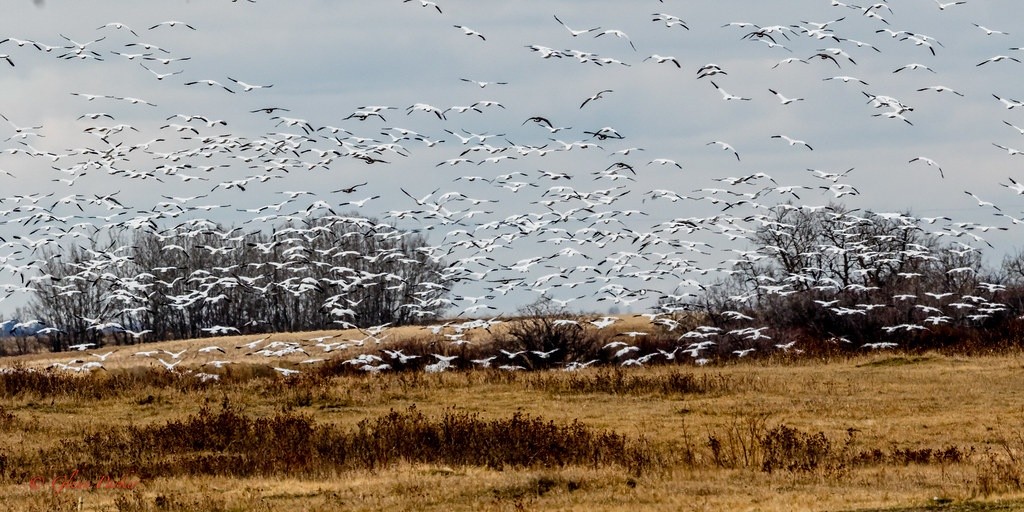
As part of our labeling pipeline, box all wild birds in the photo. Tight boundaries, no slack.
[0,0,1024,384]
